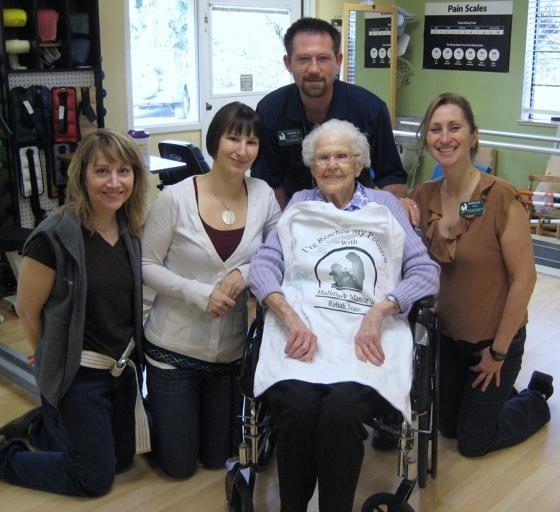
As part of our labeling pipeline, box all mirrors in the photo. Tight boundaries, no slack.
[341,4,397,131]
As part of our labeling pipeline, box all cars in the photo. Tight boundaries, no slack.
[135,48,191,121]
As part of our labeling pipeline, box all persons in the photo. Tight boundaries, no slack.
[249,16,425,231]
[404,91,555,458]
[137,101,284,485]
[243,117,443,512]
[0,126,153,501]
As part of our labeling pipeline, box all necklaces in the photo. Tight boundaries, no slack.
[207,172,244,226]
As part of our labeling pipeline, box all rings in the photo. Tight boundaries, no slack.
[413,205,421,208]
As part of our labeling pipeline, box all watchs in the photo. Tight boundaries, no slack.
[489,346,507,361]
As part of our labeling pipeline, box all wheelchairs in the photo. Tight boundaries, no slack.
[224,295,443,509]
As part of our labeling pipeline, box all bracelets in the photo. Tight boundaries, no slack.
[385,295,401,314]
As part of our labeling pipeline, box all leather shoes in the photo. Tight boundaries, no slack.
[529,371,554,401]
[0,407,45,438]
[371,416,403,450]
[0,441,28,474]
